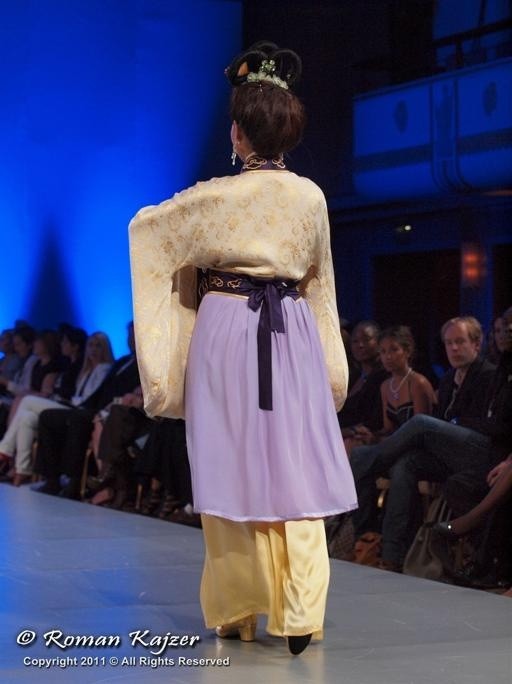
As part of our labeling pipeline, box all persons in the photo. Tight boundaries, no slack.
[0,313,510,597]
[129,80,350,655]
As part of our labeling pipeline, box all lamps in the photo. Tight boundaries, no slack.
[394,223,415,236]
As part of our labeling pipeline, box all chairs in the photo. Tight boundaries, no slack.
[30,436,512,596]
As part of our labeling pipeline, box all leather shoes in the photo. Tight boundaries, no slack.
[437,518,471,542]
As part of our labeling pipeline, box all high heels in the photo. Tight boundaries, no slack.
[215,614,257,642]
[288,633,313,655]
